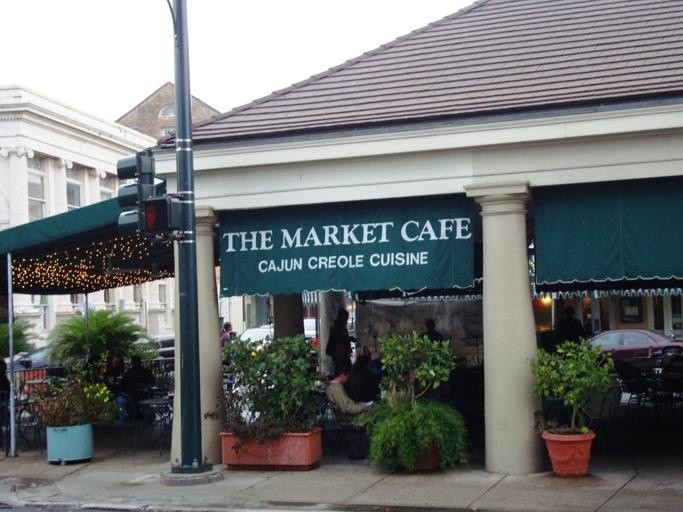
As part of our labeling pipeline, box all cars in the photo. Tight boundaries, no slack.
[582,329,683,372]
[241,317,321,352]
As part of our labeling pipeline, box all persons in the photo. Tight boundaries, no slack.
[218,322,231,351]
[78,353,108,386]
[554,305,585,345]
[325,308,355,371]
[0,358,10,401]
[414,317,444,344]
[100,351,125,389]
[109,354,154,405]
[325,367,378,430]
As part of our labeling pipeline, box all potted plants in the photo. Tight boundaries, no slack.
[367,330,468,476]
[204,332,333,471]
[34,379,121,466]
[525,336,616,478]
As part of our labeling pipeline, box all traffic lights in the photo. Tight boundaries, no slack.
[140,197,185,239]
[118,152,154,235]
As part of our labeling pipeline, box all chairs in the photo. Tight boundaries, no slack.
[108,392,156,451]
[612,346,683,418]
[4,402,43,457]
[322,392,376,457]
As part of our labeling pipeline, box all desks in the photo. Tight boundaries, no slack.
[140,398,170,455]
